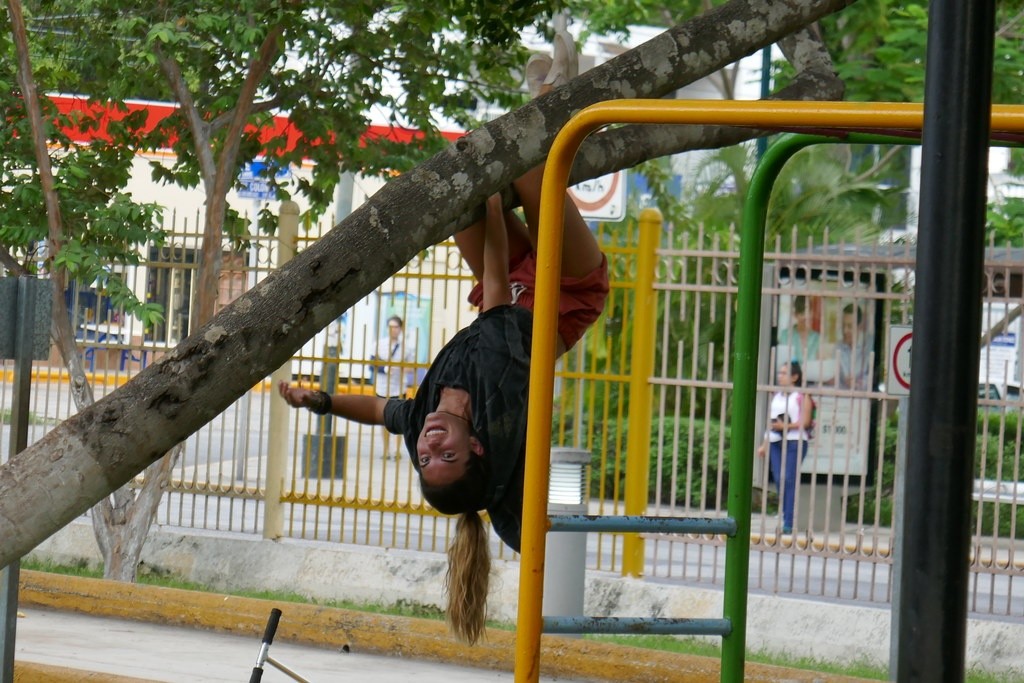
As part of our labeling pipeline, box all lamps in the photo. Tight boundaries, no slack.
[542,444,589,639]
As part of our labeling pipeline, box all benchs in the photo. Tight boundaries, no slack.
[972,480,1024,505]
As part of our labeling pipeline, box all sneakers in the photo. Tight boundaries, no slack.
[527,53,553,99]
[543,32,579,89]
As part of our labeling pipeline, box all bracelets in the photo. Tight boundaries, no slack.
[318,390,332,415]
[309,389,325,415]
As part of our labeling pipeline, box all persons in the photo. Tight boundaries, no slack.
[758,360,813,534]
[366,316,412,462]
[829,303,872,391]
[276,31,612,649]
[775,295,826,389]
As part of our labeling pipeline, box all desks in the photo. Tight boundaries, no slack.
[80,323,140,372]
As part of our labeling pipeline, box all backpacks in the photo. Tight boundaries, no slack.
[798,392,817,439]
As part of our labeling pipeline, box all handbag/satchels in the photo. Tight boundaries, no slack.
[370,343,399,374]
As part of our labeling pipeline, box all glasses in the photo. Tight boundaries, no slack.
[390,325,400,329]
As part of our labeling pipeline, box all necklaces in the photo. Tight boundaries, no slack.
[437,411,472,431]
